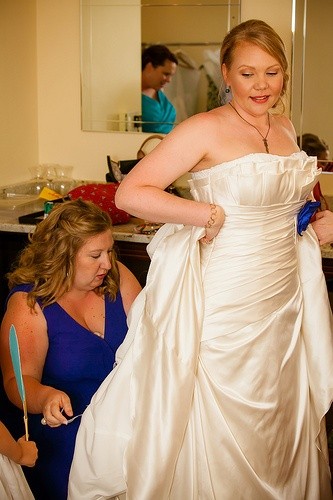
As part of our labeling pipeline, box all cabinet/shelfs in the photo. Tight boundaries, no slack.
[0,231,333,491]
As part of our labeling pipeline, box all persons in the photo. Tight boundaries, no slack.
[0,420,40,500]
[0,200,143,500]
[64,19,333,500]
[296,133,330,170]
[311,210,333,248]
[140,44,177,134]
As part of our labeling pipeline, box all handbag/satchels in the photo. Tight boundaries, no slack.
[19,185,129,226]
[107,134,185,198]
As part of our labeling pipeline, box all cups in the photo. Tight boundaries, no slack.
[30,165,74,197]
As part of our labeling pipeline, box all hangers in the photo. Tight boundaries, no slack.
[172,43,196,70]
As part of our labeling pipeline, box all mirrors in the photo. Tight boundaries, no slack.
[80,0,307,134]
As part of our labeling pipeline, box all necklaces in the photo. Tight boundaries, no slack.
[227,102,271,154]
